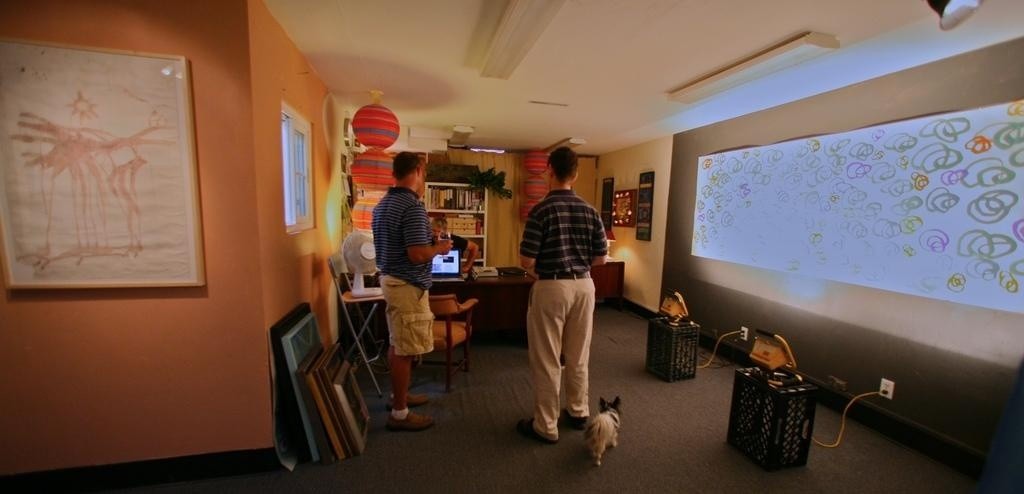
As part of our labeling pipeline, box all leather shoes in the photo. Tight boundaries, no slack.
[516,407,587,445]
[384,392,433,431]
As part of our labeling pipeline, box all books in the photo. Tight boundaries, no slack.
[425,184,485,236]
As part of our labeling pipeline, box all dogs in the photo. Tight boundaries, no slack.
[585,396,620,466]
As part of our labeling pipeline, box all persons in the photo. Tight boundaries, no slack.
[517,145,612,444]
[368,148,454,432]
[428,218,478,275]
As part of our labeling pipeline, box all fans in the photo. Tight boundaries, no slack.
[341,231,382,297]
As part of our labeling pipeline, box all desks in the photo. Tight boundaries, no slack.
[432,266,535,344]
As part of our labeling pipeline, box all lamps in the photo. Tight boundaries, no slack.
[448,125,474,145]
[481,0,565,79]
[605,230,616,261]
[544,138,586,155]
[926,0,984,31]
[667,31,841,106]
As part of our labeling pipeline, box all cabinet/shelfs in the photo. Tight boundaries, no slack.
[424,181,489,266]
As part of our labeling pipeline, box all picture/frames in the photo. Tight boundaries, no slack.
[270,302,373,467]
[0,36,206,288]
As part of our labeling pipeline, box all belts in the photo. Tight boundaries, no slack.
[539,271,592,279]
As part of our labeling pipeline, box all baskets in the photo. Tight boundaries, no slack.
[726,369,818,470]
[647,316,699,381]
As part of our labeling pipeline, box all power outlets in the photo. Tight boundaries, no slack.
[741,327,748,341]
[880,378,894,399]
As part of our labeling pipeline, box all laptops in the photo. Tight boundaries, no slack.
[431,249,464,281]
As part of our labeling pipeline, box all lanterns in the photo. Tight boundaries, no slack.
[351,190,383,231]
[522,173,547,200]
[524,149,547,175]
[519,198,539,224]
[350,102,401,150]
[350,147,396,194]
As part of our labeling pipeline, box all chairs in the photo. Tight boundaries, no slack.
[592,261,625,311]
[328,253,385,397]
[417,293,479,392]
